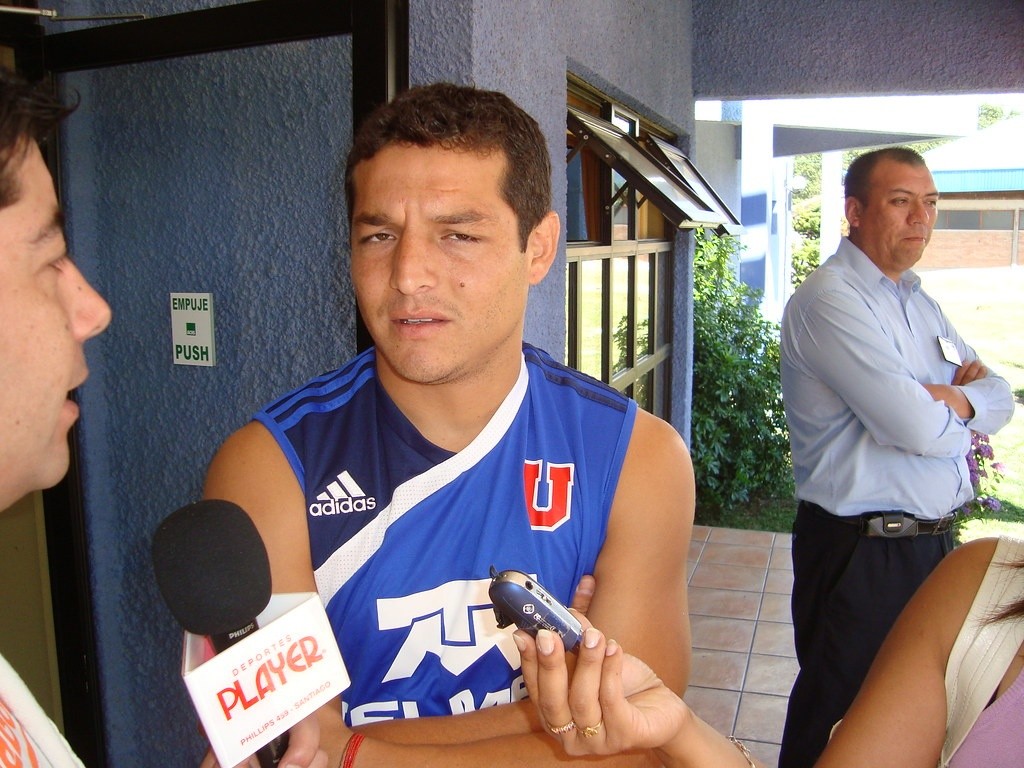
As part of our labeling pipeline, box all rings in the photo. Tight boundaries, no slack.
[545,714,603,739]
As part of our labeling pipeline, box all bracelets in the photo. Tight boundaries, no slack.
[339,731,366,768]
[726,736,756,768]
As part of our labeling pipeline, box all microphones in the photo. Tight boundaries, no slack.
[153,499,351,768]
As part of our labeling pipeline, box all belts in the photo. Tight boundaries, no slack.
[800,499,958,536]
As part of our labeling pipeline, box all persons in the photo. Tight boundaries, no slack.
[778,148,1015,768]
[205,85,696,768]
[1,64,329,768]
[512,535,1024,768]
